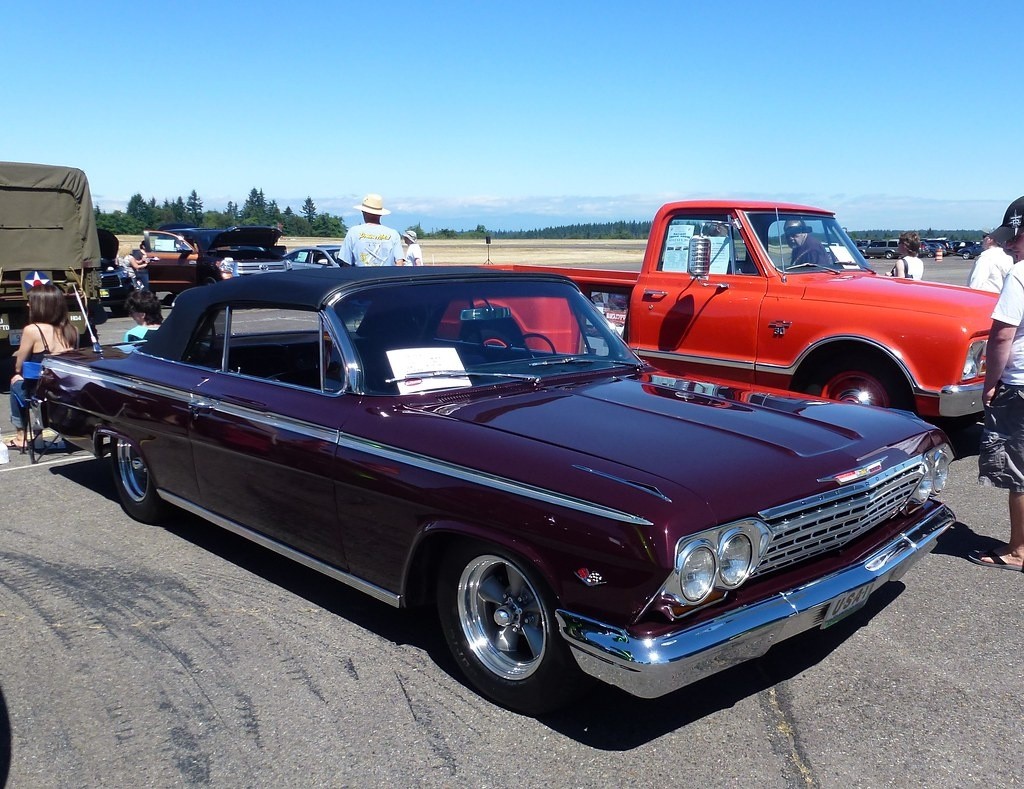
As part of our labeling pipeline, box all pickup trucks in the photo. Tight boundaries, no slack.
[434,198,1003,423]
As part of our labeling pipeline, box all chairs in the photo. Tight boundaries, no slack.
[461,306,527,368]
[12,360,60,465]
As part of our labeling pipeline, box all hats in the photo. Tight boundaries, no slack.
[988,196,1024,242]
[402,231,417,244]
[982,226,1002,246]
[351,195,391,215]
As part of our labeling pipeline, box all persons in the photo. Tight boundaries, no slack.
[403,231,423,266]
[121,241,149,289]
[2,285,79,449]
[783,220,830,265]
[337,194,406,267]
[885,232,924,280]
[967,196,1024,572]
[125,290,164,342]
[968,227,1014,294]
[708,222,739,260]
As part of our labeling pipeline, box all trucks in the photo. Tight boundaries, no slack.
[0,160,106,358]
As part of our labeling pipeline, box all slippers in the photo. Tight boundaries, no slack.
[6,440,29,450]
[968,548,1024,571]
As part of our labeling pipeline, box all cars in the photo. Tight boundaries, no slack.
[820,236,986,260]
[24,264,959,711]
[143,221,293,293]
[96,227,133,319]
[282,244,347,271]
